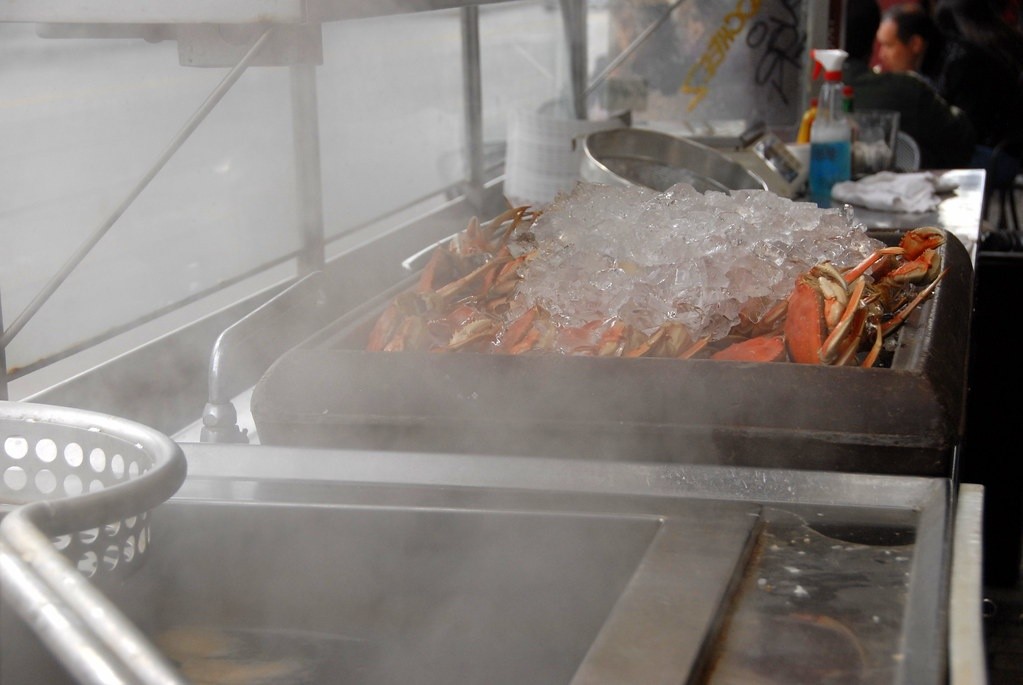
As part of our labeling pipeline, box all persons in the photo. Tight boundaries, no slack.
[828,0,1023,169]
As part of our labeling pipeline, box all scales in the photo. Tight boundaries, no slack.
[684,129,807,199]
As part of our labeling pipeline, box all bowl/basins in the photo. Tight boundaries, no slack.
[571,129,769,192]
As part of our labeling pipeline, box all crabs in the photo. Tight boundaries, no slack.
[365,205,950,368]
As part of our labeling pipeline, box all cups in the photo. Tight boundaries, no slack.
[853,109,899,178]
[765,144,810,192]
